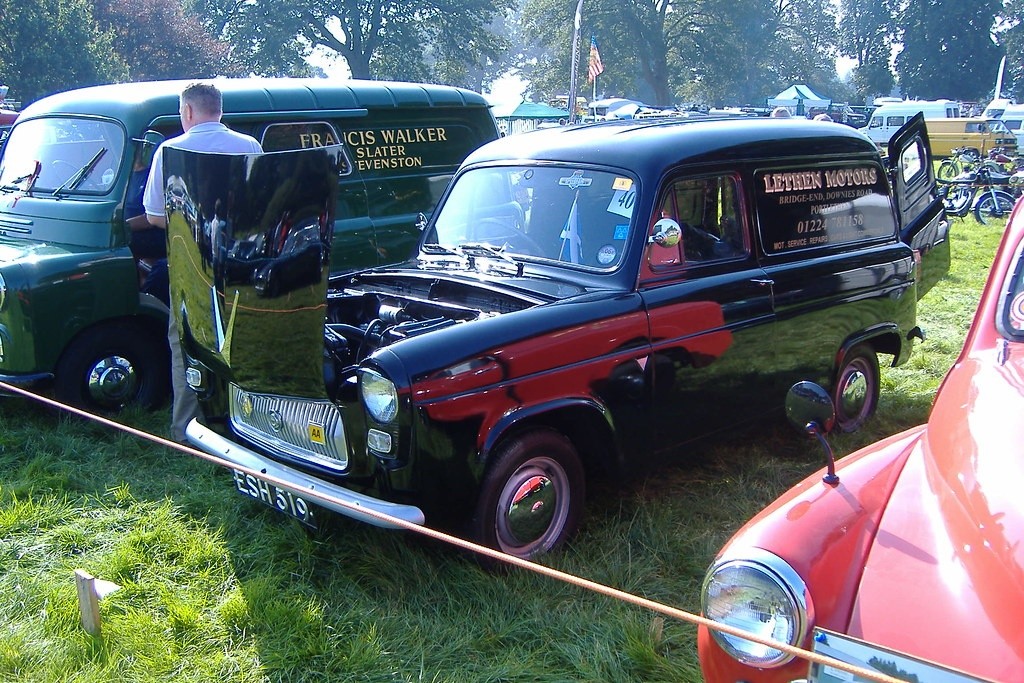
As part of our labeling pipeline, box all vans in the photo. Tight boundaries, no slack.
[867,101,962,145]
[0,78,505,418]
[926,118,1019,159]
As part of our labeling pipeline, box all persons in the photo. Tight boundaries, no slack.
[123,148,163,255]
[689,104,702,112]
[144,84,265,445]
[273,210,293,259]
[209,198,224,313]
[198,203,208,273]
[959,103,978,117]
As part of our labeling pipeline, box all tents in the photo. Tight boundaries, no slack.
[767,85,834,115]
[493,99,582,136]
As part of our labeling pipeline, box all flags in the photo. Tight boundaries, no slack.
[588,35,606,83]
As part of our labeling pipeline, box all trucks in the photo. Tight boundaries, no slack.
[0,110,111,187]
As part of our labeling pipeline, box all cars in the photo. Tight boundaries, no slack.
[697,193,1024,681]
[978,98,1024,157]
[166,188,328,297]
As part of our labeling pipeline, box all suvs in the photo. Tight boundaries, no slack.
[807,105,866,128]
[222,110,953,573]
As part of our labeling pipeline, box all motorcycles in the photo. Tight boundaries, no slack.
[933,144,1024,225]
